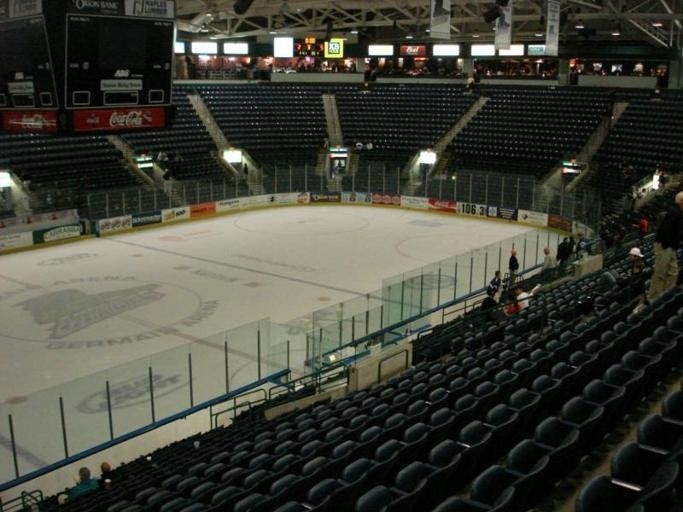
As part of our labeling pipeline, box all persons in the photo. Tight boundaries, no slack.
[462,81,480,97]
[138,144,153,158]
[173,150,184,163]
[163,168,171,193]
[466,166,683,319]
[56,466,100,504]
[96,461,114,490]
[156,147,170,162]
[179,57,667,81]
